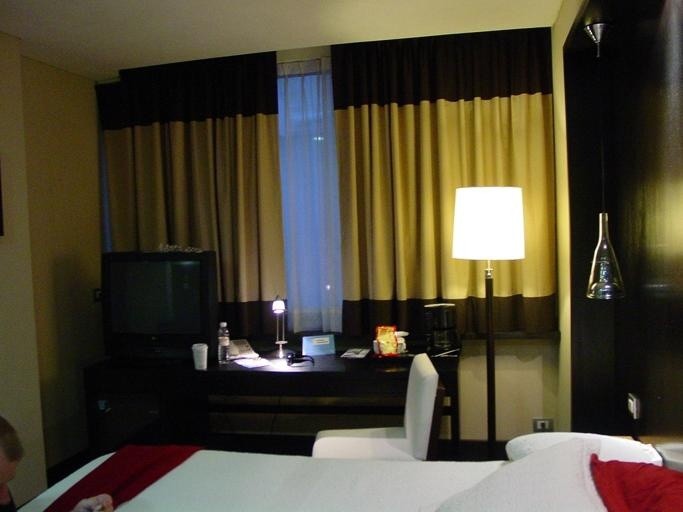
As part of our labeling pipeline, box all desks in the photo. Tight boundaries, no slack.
[94,338,461,460]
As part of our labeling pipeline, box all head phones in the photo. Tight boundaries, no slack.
[288,352,314,365]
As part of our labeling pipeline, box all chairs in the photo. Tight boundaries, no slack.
[311,352,440,460]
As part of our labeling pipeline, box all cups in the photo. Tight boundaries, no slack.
[192,343,208,371]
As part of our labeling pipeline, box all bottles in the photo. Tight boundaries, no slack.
[586,212,625,300]
[218,322,230,364]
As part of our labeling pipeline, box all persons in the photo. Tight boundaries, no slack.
[0,416,115,512]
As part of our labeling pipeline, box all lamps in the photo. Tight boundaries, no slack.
[272,296,288,358]
[583,21,627,302]
[450,186,526,461]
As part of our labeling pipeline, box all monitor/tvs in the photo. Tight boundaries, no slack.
[100,248,218,360]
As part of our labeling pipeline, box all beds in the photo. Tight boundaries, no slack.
[14,443,682,512]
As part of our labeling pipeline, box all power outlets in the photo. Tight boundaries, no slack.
[533,420,553,433]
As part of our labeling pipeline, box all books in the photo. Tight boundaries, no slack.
[224,338,259,361]
[339,347,371,359]
[438,352,460,359]
[428,345,460,359]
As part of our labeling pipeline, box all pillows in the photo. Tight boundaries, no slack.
[441,438,609,512]
[589,453,682,512]
[505,433,662,467]
